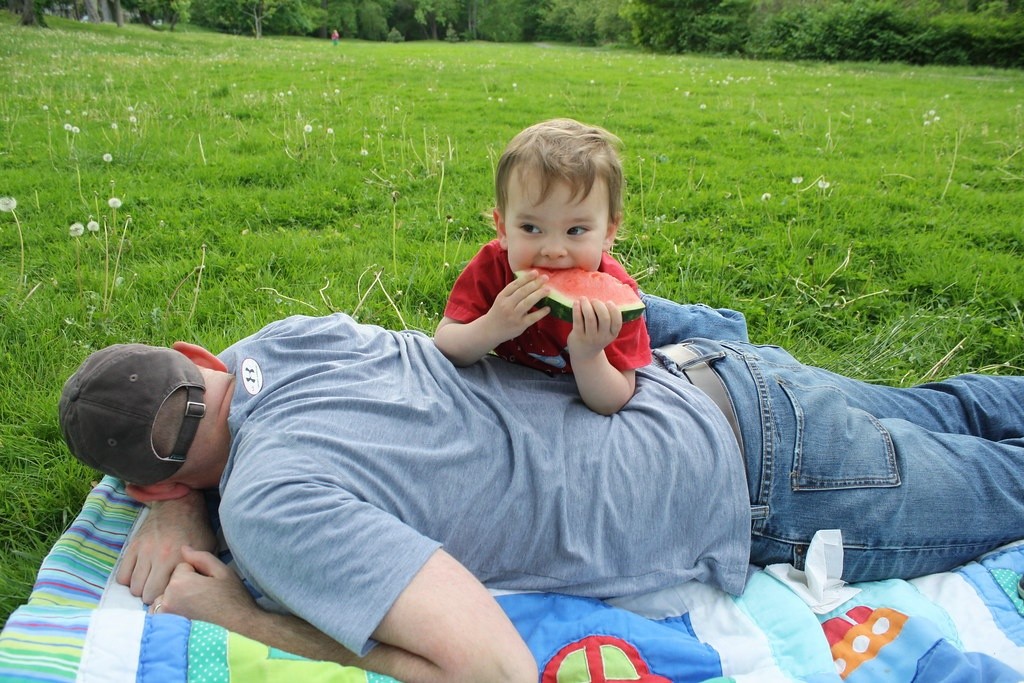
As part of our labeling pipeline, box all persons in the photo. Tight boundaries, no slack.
[57,312,1024,682]
[434,117,750,414]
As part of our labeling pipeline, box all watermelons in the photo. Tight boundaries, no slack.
[513,267,646,323]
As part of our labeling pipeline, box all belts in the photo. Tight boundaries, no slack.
[654,343,745,460]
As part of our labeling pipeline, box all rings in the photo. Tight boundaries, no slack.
[154,604,160,614]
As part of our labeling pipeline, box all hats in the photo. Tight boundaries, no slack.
[58,344,206,484]
